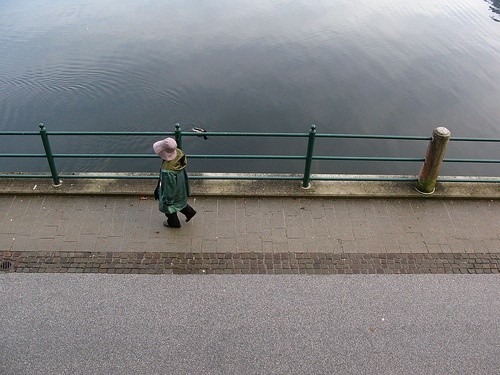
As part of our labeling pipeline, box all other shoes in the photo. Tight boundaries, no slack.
[163,221,181,228]
[185,211,196,222]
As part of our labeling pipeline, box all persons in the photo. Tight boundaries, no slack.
[152,137,197,228]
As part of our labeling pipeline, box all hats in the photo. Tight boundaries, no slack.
[153,138,177,161]
